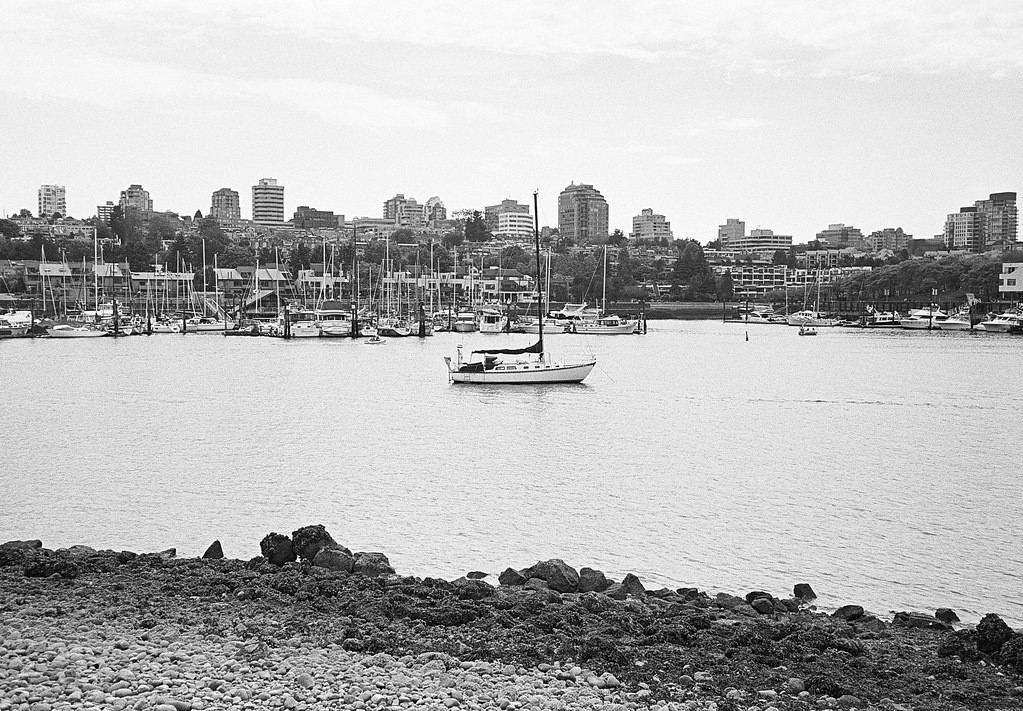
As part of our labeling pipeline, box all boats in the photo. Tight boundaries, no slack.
[739,311,786,322]
[936,315,971,330]
[981,307,1023,331]
[973,322,987,330]
[865,305,900,324]
[798,327,818,335]
[899,310,945,328]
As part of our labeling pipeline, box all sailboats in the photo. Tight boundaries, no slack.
[570,244,637,334]
[0,228,456,337]
[442,190,598,384]
[455,264,477,332]
[479,254,503,333]
[518,246,564,334]
[788,270,840,327]
